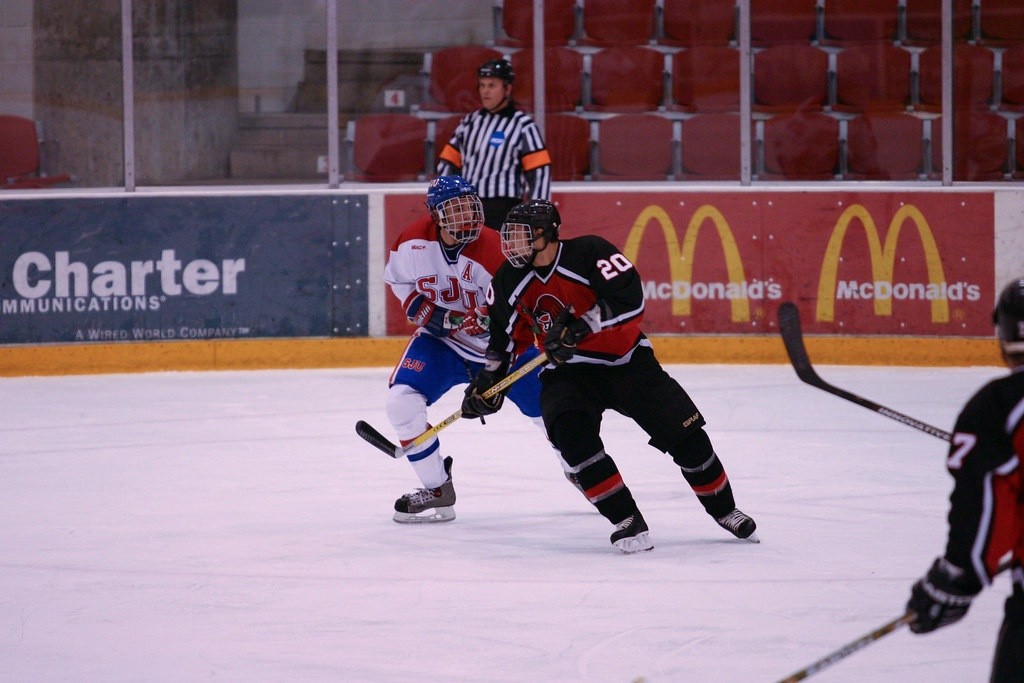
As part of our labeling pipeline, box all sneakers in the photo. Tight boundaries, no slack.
[715,507,761,543]
[610,506,654,554]
[393,455,457,524]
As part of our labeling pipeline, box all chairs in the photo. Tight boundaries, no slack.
[0,115,70,190]
[750,0,1024,183]
[667,49,741,112]
[597,113,673,181]
[576,0,657,45]
[354,115,428,181]
[681,114,740,180]
[498,0,577,46]
[512,49,582,112]
[545,114,591,180]
[657,0,737,46]
[426,115,465,182]
[421,47,503,114]
[585,47,664,112]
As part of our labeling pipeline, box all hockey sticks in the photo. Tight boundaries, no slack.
[778,559,1010,682]
[354,353,549,459]
[778,303,954,443]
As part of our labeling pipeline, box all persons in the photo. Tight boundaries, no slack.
[461,199,757,542]
[382,175,590,514]
[905,276,1024,683]
[437,57,551,232]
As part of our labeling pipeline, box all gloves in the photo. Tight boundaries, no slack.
[906,557,975,633]
[543,316,592,367]
[407,295,465,338]
[461,368,504,425]
[462,305,491,338]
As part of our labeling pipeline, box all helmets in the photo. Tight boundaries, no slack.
[991,278,1024,359]
[478,60,515,87]
[424,176,485,244]
[500,197,561,268]
